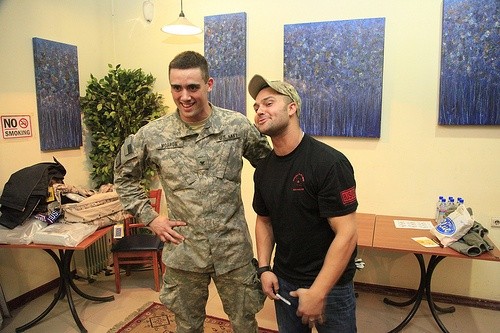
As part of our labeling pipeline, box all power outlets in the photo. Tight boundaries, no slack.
[490,216,500,227]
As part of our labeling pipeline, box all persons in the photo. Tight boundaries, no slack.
[248,74,358,333]
[114,52,272,333]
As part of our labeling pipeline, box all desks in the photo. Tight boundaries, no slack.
[0,224,114,333]
[373,215,500,333]
[354,211,377,247]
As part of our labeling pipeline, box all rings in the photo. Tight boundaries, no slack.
[309,319,314,323]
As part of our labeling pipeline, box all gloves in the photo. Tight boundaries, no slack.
[355,258,366,271]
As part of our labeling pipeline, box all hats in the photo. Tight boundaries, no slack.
[248,74,302,116]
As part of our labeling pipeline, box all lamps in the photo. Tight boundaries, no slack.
[161,0,204,36]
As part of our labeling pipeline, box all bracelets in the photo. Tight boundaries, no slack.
[257,266,272,279]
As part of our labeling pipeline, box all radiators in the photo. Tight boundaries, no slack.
[72,227,114,283]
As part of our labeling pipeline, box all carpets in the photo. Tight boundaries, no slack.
[106,302,280,333]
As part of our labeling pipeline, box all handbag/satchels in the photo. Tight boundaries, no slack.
[63,192,130,230]
[430,204,474,248]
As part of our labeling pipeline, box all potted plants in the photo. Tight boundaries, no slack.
[75,63,168,268]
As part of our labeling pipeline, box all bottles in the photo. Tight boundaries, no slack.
[447,196,456,207]
[435,196,447,224]
[456,197,464,207]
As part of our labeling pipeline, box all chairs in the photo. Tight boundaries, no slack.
[110,189,166,294]
